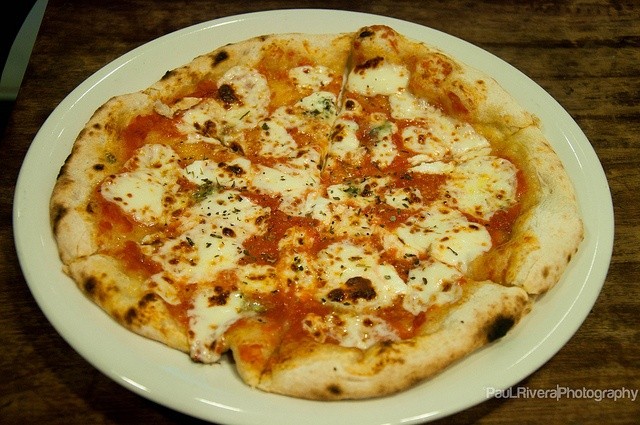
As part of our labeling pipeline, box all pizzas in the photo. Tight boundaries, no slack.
[48,23,586,404]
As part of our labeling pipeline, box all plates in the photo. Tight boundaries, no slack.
[13,9,614,425]
